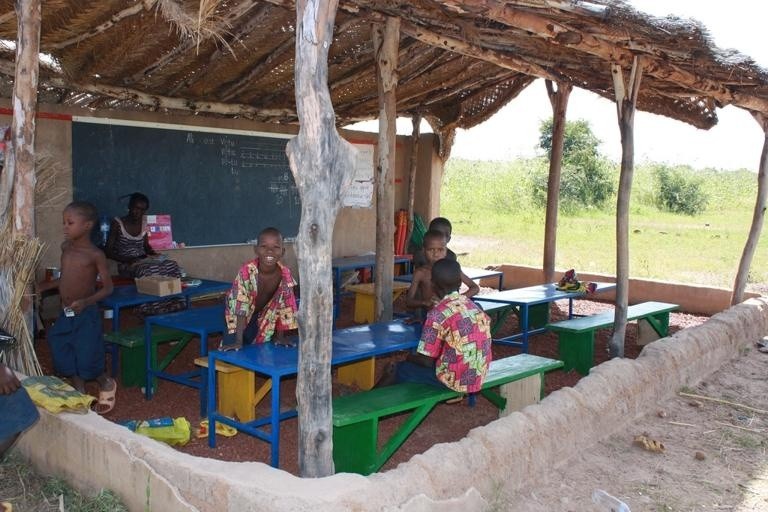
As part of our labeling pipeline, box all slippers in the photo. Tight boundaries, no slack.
[96,379,117,415]
[196,416,238,438]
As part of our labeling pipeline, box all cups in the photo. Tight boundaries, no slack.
[45,268,59,280]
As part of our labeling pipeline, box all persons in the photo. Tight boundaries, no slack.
[1,361,41,512]
[37,199,117,415]
[428,216,457,262]
[396,258,493,405]
[217,226,300,352]
[104,192,186,316]
[406,231,480,320]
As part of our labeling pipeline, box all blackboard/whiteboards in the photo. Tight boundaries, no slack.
[71,116,302,251]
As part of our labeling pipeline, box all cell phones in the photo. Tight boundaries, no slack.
[64,307,75,318]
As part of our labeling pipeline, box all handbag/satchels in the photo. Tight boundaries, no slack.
[20,375,96,414]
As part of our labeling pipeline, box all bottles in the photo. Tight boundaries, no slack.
[99,213,110,245]
